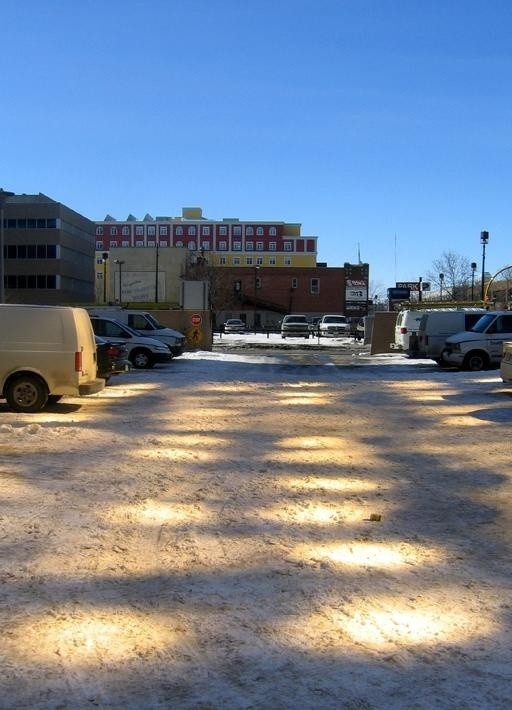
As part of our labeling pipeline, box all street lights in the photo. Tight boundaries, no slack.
[479,228,490,300]
[469,260,478,300]
[253,263,263,333]
[113,258,125,305]
[101,250,110,302]
[440,273,445,302]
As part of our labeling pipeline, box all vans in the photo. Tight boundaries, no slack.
[390,300,512,386]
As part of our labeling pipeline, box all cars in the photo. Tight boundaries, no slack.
[221,317,247,335]
[279,315,371,339]
[1,300,190,416]
